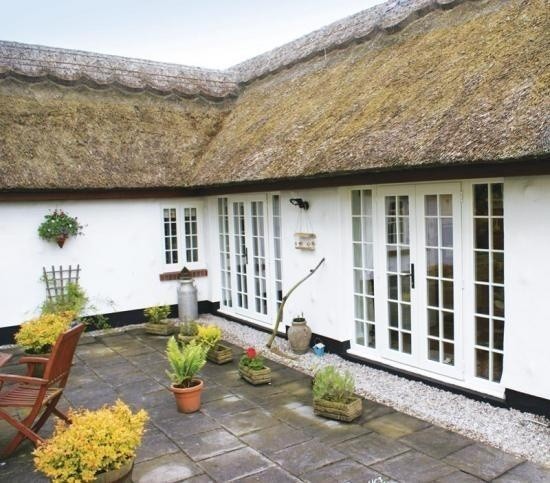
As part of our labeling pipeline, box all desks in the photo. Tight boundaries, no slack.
[0,352,12,371]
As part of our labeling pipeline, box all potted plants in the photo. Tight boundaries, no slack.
[143,305,233,364]
[163,336,210,414]
[311,365,362,423]
[33,399,150,483]
[239,347,271,386]
[15,311,76,378]
[37,207,88,248]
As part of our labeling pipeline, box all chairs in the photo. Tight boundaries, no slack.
[1,322,86,457]
